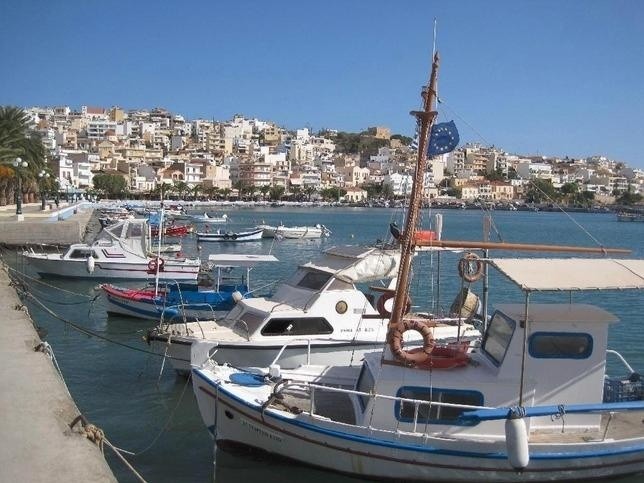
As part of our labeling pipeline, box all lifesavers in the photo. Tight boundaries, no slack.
[148,258,165,271]
[390,320,435,364]
[459,252,484,282]
[377,291,412,318]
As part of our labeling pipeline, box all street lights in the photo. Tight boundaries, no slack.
[11,156,27,223]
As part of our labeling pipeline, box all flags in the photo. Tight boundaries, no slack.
[428,119,459,157]
[410,116,421,149]
